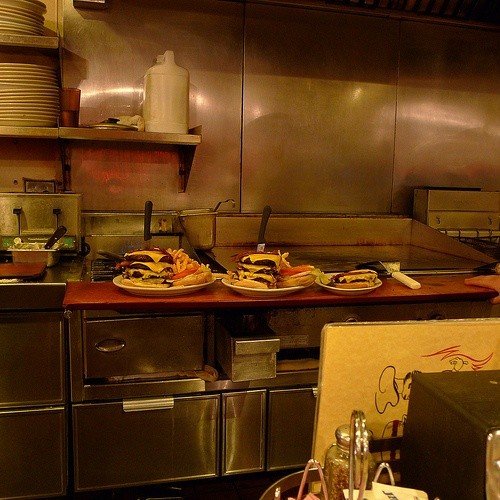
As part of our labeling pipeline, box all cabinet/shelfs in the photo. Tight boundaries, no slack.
[0,309,320,500]
[0,33,206,194]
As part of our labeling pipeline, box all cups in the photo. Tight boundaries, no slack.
[61,88,81,128]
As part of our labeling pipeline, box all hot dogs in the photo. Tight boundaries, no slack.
[277,264,321,288]
[170,263,212,286]
[330,269,378,288]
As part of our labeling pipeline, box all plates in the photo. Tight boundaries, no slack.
[113,274,216,296]
[0,63,61,127]
[221,273,305,298]
[0,0,48,36]
[314,272,383,295]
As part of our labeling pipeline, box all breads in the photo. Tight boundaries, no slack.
[230,280,268,289]
[122,277,170,288]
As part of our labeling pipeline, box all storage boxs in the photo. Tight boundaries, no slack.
[215,321,281,382]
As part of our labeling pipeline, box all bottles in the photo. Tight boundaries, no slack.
[320,424,377,500]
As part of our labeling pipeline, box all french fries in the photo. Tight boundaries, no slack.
[165,247,209,272]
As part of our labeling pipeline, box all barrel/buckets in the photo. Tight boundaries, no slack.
[143,50,190,134]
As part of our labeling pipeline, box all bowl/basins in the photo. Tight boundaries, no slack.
[6,243,68,267]
